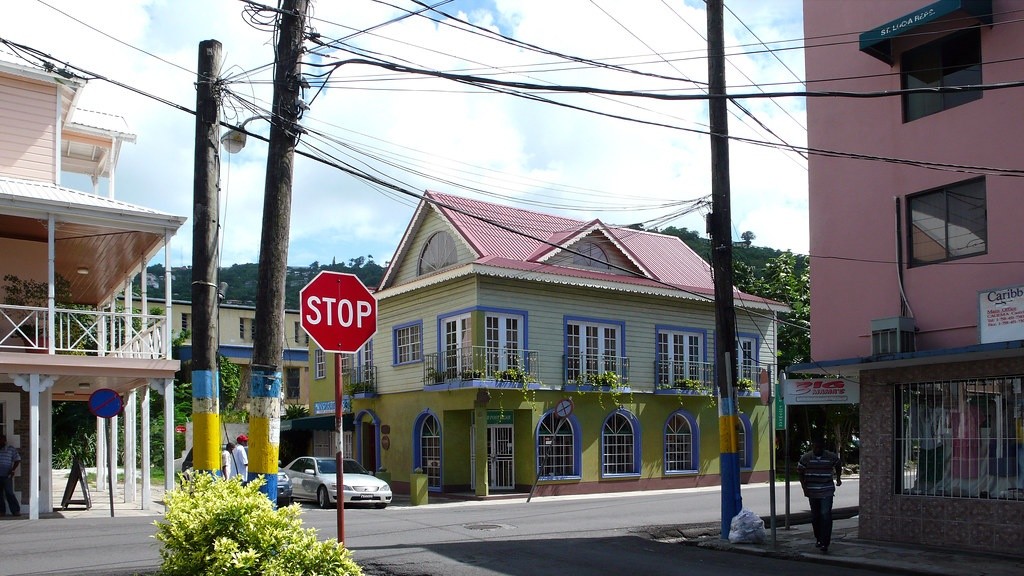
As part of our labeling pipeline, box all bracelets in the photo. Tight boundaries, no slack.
[12,471,15,472]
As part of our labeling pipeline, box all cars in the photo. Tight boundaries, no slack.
[282,456,392,509]
[242,445,292,511]
[182,443,227,472]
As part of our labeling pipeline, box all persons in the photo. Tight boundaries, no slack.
[231,433,248,481]
[796,440,842,551]
[0,434,22,517]
[221,443,235,482]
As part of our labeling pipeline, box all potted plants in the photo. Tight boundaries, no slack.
[657,378,717,408]
[419,367,446,384]
[458,368,482,382]
[735,377,755,395]
[344,381,373,397]
[574,372,633,413]
[0,272,71,353]
[494,367,544,423]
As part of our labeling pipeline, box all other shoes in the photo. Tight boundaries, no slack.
[14,511,21,516]
[815,539,822,548]
[820,545,828,551]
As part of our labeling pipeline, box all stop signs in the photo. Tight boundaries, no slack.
[299,271,377,354]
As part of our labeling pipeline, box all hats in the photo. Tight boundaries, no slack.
[237,435,249,444]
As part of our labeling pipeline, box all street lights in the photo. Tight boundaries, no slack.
[221,115,294,508]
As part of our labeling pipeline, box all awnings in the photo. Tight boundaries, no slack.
[859,0,993,66]
[281,412,355,432]
